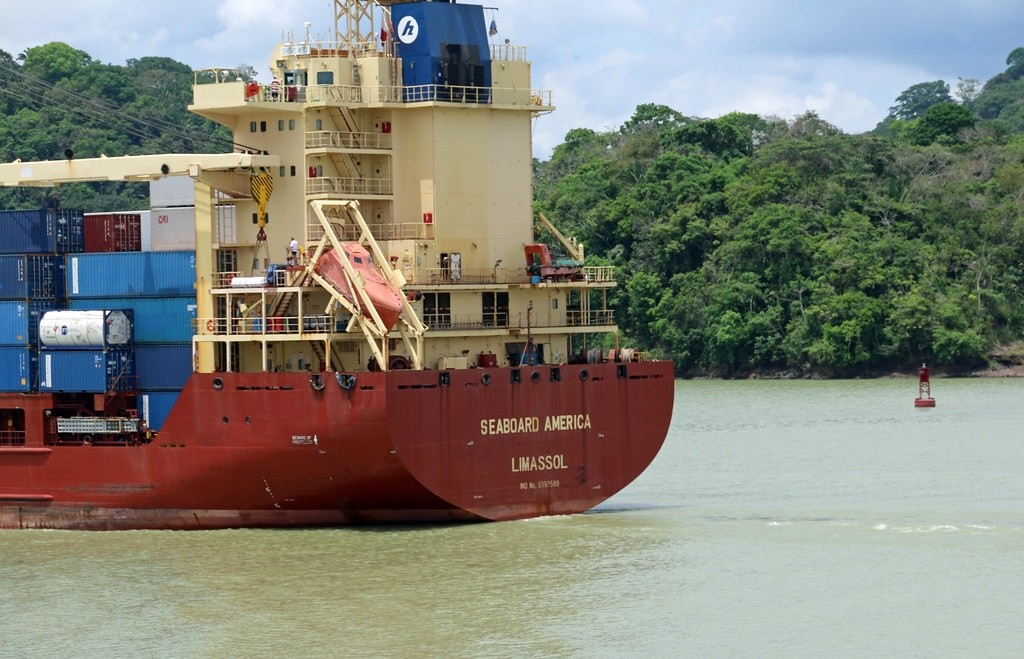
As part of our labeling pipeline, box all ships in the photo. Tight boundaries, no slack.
[0,0,677,530]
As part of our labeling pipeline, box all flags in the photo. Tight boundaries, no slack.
[487,20,498,36]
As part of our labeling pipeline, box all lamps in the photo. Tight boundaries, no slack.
[278,62,282,67]
[321,61,326,66]
[220,70,229,83]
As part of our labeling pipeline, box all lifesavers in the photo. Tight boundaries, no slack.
[246,82,259,96]
[206,319,216,332]
[227,273,235,285]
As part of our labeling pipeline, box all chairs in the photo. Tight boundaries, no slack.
[248,85,296,102]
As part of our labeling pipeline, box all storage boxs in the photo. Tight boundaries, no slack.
[68,297,196,345]
[84,214,141,253]
[66,250,196,299]
[150,205,236,250]
[0,210,84,393]
[135,391,182,432]
[39,345,192,393]
[149,175,231,207]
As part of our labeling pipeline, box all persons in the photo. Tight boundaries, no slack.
[289,237,298,265]
